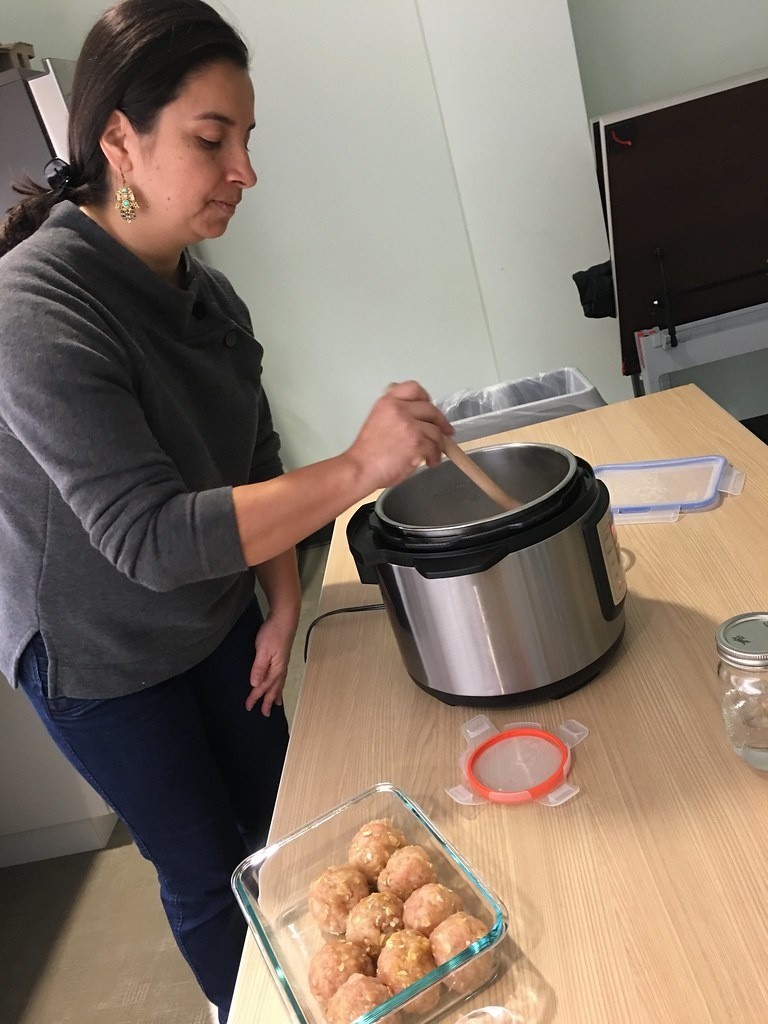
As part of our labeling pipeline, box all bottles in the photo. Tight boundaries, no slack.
[717,613,768,772]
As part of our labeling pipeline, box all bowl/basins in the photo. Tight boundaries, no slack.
[230,782,506,1024]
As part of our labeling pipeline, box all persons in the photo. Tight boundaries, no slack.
[0,0,454,1023]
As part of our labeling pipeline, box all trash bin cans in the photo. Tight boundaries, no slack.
[433,366,609,448]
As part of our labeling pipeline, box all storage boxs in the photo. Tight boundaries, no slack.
[231,781,509,1024]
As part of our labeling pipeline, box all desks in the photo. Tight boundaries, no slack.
[226,383,768,1024]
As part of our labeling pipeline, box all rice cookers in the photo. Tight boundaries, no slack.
[345,442,628,709]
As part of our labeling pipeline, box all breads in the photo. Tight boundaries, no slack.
[306,819,492,1024]
